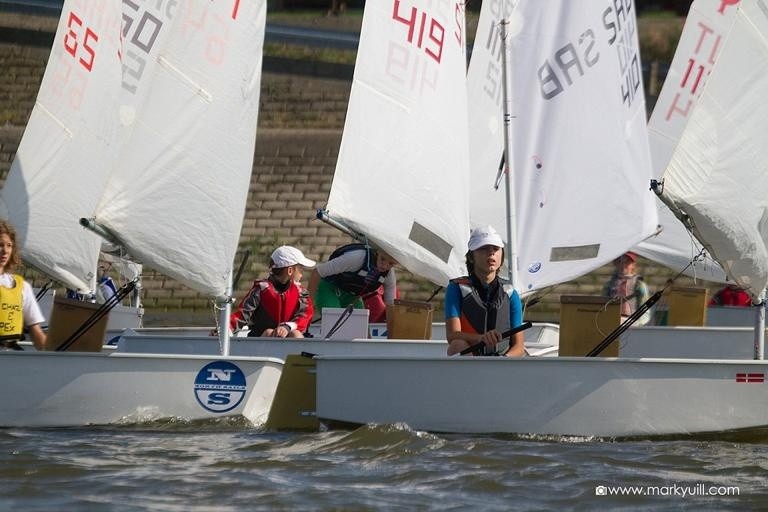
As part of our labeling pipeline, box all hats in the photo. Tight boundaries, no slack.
[268,245,317,268]
[613,251,638,261]
[467,225,505,251]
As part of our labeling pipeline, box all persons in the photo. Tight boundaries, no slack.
[208,245,319,339]
[443,225,526,358]
[706,277,752,308]
[0,215,48,355]
[304,242,401,328]
[602,250,650,328]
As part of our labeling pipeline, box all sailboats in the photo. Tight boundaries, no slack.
[0,0,285,434]
[306,0,767,441]
